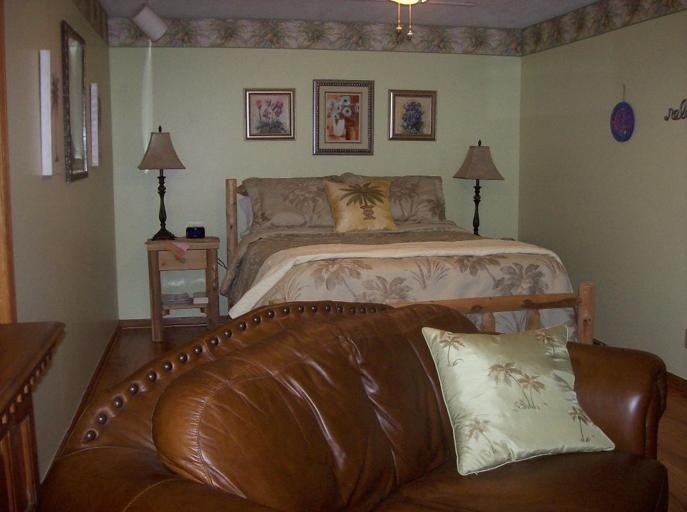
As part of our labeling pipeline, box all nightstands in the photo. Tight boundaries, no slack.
[145,237,220,343]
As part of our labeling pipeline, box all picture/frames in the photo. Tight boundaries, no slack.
[313,79,374,155]
[389,89,436,141]
[244,87,297,140]
[60,19,89,184]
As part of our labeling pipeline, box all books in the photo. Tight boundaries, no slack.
[192,289,208,305]
[160,292,192,305]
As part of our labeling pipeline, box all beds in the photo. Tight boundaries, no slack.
[226,173,595,347]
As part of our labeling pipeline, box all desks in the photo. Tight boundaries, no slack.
[1,319,65,511]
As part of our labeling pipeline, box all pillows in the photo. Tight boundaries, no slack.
[340,173,447,224]
[423,324,617,474]
[323,179,397,233]
[243,175,341,234]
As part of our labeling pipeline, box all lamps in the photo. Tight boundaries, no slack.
[452,140,504,236]
[137,125,186,241]
[132,2,169,43]
[392,0,428,40]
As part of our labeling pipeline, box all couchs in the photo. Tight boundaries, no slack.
[41,301,671,510]
